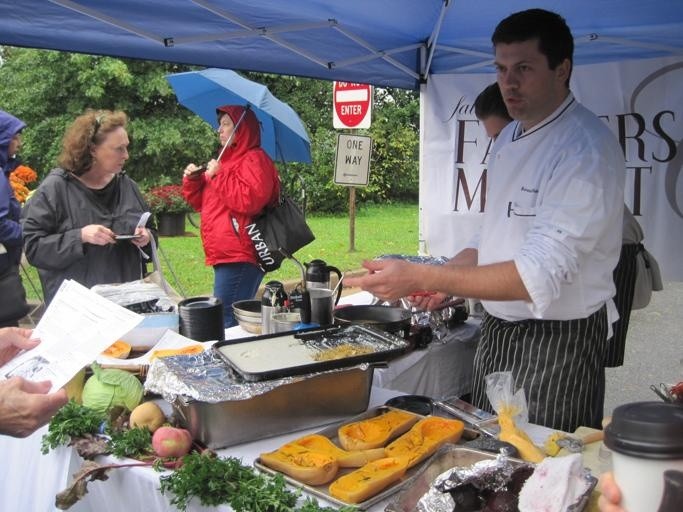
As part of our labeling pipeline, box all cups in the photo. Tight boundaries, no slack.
[604,402,683,512]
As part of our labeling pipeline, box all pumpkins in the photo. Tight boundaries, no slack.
[288,433,387,468]
[260,442,338,487]
[383,415,465,471]
[328,454,409,506]
[102,340,132,359]
[338,409,420,452]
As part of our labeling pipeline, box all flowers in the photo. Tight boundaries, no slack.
[143,184,196,213]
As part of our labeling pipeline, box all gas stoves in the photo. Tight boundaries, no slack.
[393,324,431,357]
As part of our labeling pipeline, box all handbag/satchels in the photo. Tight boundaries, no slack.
[142,270,185,305]
[243,192,316,273]
[0,266,30,327]
[89,280,178,315]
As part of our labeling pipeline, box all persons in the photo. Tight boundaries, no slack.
[0,325,70,438]
[340,8,627,435]
[473,80,665,369]
[0,110,38,327]
[182,104,281,331]
[21,106,159,309]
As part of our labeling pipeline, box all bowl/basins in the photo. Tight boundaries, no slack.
[234,311,262,323]
[271,312,302,333]
[232,300,262,317]
[235,317,262,334]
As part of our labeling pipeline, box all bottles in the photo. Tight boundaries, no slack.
[262,280,289,334]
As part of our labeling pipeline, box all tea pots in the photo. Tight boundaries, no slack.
[303,259,343,326]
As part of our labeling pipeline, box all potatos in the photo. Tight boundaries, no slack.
[129,401,166,435]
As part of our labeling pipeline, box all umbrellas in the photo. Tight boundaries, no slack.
[161,66,313,165]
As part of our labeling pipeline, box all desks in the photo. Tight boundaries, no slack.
[0,295,619,512]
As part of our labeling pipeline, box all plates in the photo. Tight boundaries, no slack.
[178,296,224,343]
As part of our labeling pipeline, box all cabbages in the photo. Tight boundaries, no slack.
[82,361,147,422]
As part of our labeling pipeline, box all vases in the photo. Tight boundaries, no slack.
[156,213,186,235]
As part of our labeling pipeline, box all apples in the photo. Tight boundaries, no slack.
[151,426,193,459]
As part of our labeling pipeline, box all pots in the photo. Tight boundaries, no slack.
[334,298,465,332]
[384,395,480,441]
